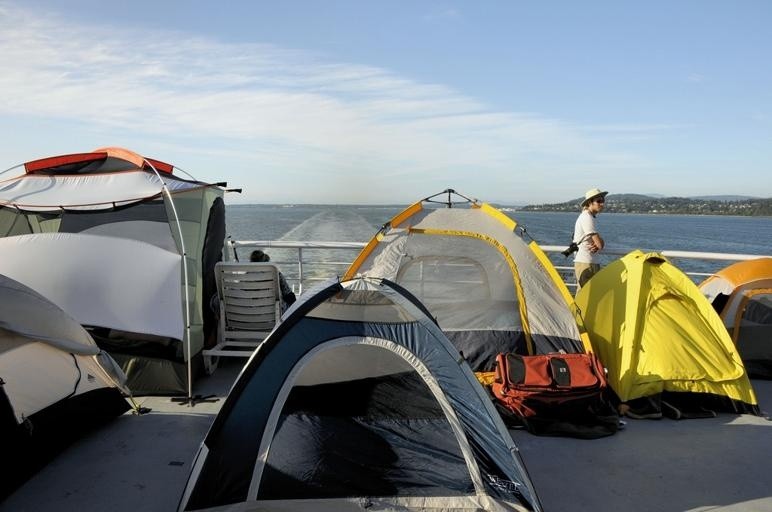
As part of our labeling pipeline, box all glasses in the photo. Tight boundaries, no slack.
[597,199,605,204]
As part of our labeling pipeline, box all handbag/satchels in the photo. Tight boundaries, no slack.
[484,350,620,441]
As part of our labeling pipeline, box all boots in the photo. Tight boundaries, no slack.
[626,395,717,420]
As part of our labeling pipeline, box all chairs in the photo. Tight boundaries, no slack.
[202,261,289,376]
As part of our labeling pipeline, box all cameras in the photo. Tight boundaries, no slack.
[561,241,579,260]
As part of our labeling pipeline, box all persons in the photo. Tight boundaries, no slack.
[238,249,297,315]
[572,189,609,298]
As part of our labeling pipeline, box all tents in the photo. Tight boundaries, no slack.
[573,249,762,421]
[176,274,545,511]
[696,258,772,382]
[339,188,628,440]
[0,145,244,408]
[0,273,152,500]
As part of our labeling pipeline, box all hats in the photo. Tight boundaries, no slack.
[580,189,608,207]
